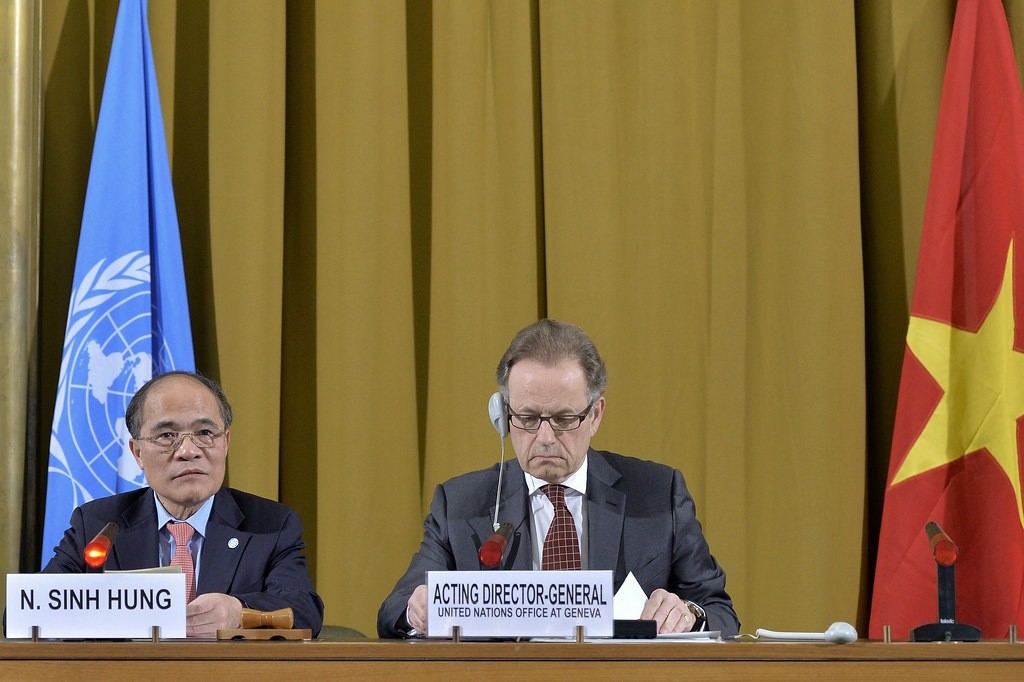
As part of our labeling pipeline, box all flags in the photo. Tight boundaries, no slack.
[40,0,197,569]
[869,0,1024,641]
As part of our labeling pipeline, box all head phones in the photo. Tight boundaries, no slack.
[488,392,509,438]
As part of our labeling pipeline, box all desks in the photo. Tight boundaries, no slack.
[0,635,1024,682]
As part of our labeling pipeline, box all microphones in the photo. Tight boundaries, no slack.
[479,521,513,567]
[925,521,958,567]
[82,522,119,568]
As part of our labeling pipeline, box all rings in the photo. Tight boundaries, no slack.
[681,613,690,623]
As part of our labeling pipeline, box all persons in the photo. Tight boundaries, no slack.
[376,319,742,641]
[42,370,325,640]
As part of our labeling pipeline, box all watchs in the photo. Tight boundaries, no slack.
[682,599,706,632]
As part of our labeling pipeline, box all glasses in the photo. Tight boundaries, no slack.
[132,429,225,448]
[506,400,596,431]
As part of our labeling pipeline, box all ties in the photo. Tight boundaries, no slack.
[539,484,581,570]
[165,522,198,605]
